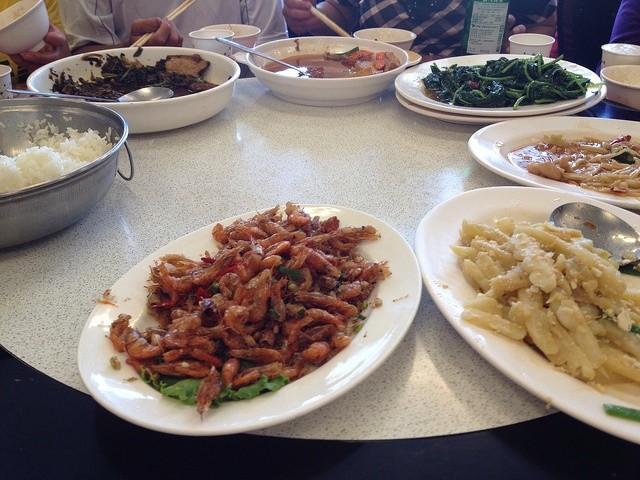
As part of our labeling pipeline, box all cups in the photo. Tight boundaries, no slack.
[509,33,555,56]
[189,29,234,56]
[0,64,14,99]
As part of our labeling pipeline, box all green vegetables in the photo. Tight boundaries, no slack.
[422,53,600,110]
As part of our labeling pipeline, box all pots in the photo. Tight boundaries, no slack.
[1,99,134,249]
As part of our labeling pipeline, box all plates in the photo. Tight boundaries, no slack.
[394,53,602,115]
[467,116,640,210]
[395,83,608,124]
[415,186,640,445]
[77,204,423,436]
[406,51,422,67]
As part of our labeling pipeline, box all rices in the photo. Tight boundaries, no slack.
[1,111,121,198]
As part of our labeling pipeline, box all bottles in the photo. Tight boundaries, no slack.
[461,0,511,55]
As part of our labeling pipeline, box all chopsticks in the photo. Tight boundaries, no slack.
[309,5,351,37]
[128,0,195,49]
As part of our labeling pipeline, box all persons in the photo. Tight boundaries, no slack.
[61,1,290,51]
[0,0,70,77]
[282,0,557,56]
[594,0,639,73]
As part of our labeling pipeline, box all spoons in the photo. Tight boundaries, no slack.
[549,202,640,267]
[6,87,174,102]
[215,36,309,76]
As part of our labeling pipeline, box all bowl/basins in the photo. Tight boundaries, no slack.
[202,23,261,54]
[0,1,50,55]
[601,43,640,68]
[233,35,408,106]
[353,28,417,51]
[26,46,241,134]
[600,65,640,108]
